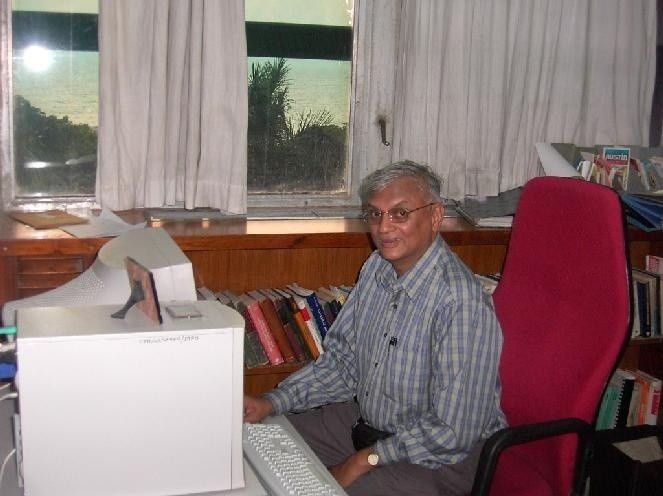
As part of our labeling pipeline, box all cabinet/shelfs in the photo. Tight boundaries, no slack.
[586,226,663,494]
[0,211,512,396]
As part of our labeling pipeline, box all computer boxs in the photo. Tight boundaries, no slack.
[16,301,246,494]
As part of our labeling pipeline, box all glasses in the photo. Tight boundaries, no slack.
[362,203,433,223]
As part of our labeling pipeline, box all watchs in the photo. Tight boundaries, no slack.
[367,443,382,469]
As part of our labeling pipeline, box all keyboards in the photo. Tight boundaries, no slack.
[244,423,350,496]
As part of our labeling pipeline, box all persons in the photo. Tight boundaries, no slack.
[242,157,511,496]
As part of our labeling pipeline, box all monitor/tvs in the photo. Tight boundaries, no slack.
[2,228,198,345]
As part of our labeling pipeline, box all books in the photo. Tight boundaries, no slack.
[197,282,356,369]
[575,141,663,435]
[475,271,503,294]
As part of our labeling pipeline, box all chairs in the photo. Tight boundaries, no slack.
[471,176,635,496]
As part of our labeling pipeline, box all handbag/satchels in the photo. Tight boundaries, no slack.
[352,423,394,452]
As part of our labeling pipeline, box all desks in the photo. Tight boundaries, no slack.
[0,384,349,496]
